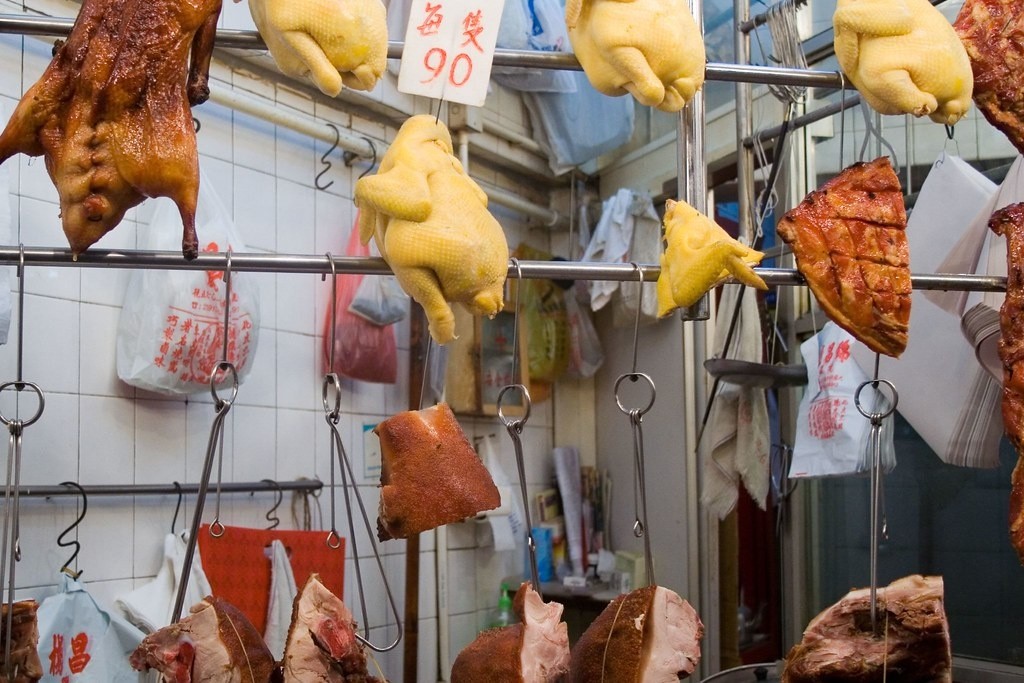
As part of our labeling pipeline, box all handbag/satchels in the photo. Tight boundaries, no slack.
[491,0,634,176]
[510,242,570,383]
[321,209,398,385]
[566,285,604,379]
[115,160,259,397]
[347,237,411,327]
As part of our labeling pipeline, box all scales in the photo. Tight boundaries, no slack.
[690,87,818,453]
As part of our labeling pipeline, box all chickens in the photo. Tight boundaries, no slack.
[0,0,974,348]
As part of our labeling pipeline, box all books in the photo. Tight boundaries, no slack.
[534,465,612,580]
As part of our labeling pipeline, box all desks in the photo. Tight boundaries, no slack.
[502,574,610,649]
